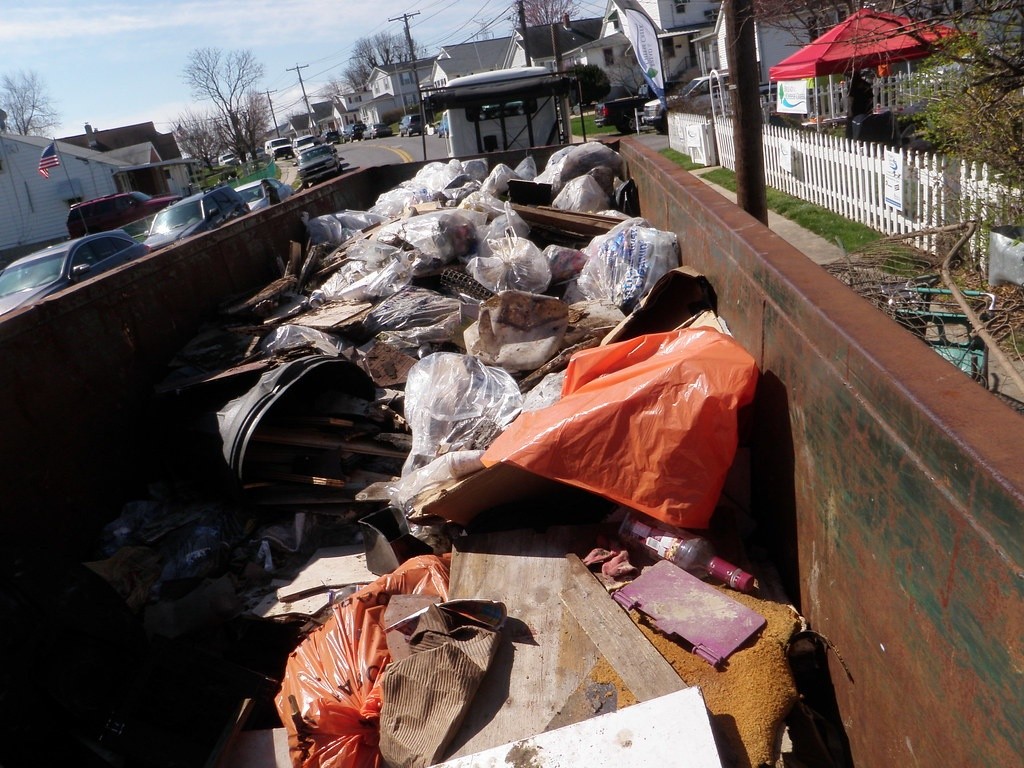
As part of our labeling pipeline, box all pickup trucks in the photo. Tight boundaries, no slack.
[643,72,777,135]
[592,79,690,134]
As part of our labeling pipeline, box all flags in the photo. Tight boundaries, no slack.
[38,143,60,179]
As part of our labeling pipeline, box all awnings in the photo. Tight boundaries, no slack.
[111,158,200,178]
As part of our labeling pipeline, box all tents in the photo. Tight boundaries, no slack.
[768,6,978,81]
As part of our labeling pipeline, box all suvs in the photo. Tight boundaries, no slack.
[293,144,342,190]
[0,227,152,319]
[292,135,323,161]
[263,138,296,162]
[341,121,368,144]
[66,189,185,238]
[217,152,240,168]
[144,182,253,253]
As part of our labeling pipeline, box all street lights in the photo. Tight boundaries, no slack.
[391,44,427,126]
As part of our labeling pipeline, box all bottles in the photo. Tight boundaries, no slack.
[617,508,755,592]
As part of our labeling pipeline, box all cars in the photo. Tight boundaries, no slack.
[361,123,394,141]
[320,130,342,144]
[435,112,449,139]
[247,147,266,163]
[234,177,296,212]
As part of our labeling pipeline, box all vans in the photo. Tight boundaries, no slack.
[397,113,426,137]
